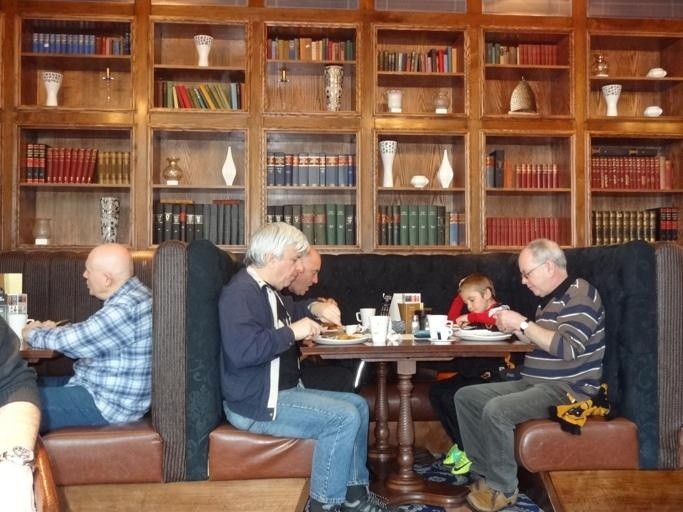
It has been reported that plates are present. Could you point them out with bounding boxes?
[428,339,455,342]
[307,326,369,345]
[450,324,475,329]
[456,329,513,342]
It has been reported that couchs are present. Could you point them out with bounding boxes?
[190,243,316,511]
[512,243,674,509]
[40,249,183,505]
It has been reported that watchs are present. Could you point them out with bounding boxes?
[520,317,531,335]
[0,445,36,473]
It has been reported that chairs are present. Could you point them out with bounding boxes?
[26,441,67,508]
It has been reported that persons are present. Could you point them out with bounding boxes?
[215,217,397,511]
[453,239,608,512]
[283,249,385,482]
[413,271,527,487]
[0,316,44,511]
[21,243,153,432]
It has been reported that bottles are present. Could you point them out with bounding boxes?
[591,53,611,76]
[29,217,52,245]
[162,157,183,185]
[221,146,236,185]
[437,149,454,188]
[411,315,420,334]
[432,91,450,114]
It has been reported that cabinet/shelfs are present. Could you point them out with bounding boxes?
[0,1,681,248]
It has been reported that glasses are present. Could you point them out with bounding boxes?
[519,260,549,280]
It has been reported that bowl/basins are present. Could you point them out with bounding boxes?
[409,176,429,188]
[645,68,667,79]
[643,106,663,118]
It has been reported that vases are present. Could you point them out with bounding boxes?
[218,143,239,188]
[599,78,623,118]
[591,46,614,81]
[319,62,347,114]
[29,213,55,245]
[188,31,215,69]
[384,84,410,116]
[158,154,188,187]
[429,87,451,117]
[409,175,430,190]
[643,66,669,80]
[93,196,120,246]
[375,139,400,190]
[642,105,664,120]
[436,148,456,190]
[39,67,67,110]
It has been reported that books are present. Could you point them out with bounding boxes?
[592,208,677,246]
[378,205,466,246]
[591,148,674,190]
[158,78,245,110]
[487,217,561,246]
[485,43,557,65]
[27,145,131,184]
[378,47,457,73]
[266,38,353,60]
[267,204,355,245]
[486,150,560,188]
[32,32,130,55]
[267,152,356,187]
[154,199,245,245]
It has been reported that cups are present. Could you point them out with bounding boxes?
[379,139,396,187]
[356,307,376,326]
[368,315,389,345]
[601,84,622,117]
[385,91,402,113]
[7,314,35,352]
[426,314,453,338]
[40,71,63,106]
[98,197,120,243]
[322,65,344,111]
[192,35,213,66]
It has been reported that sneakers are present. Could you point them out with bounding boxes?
[466,479,520,512]
[304,497,341,512]
[451,449,473,476]
[442,442,465,466]
[340,490,393,512]
